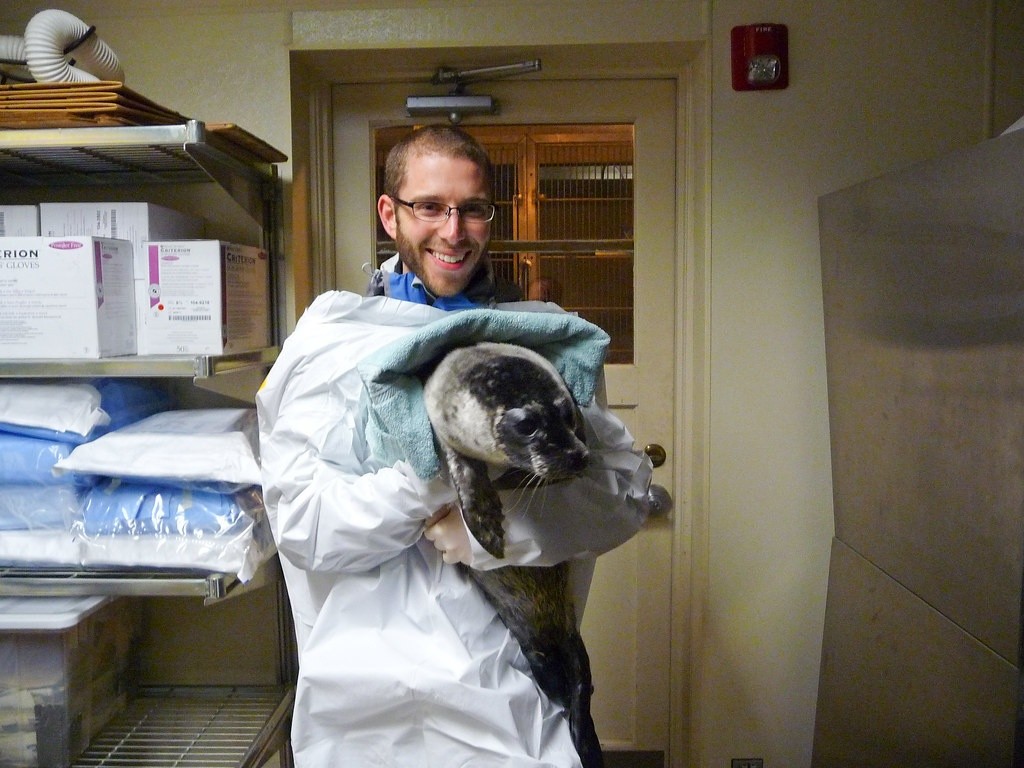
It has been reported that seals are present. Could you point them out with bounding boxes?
[423,343,605,768]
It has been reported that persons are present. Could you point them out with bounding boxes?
[258,124,651,768]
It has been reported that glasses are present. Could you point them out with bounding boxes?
[390,195,500,223]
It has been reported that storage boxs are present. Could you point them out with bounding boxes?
[40,202,203,326]
[0,205,40,237]
[142,240,273,354]
[0,595,139,768]
[0,236,138,359]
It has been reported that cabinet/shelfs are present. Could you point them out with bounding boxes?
[0,7,291,768]
